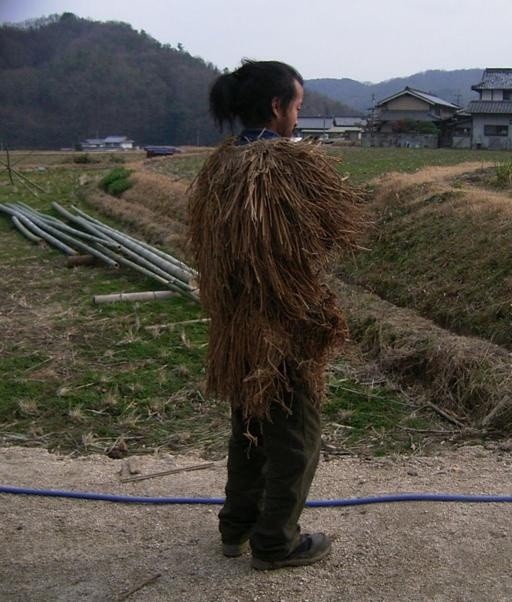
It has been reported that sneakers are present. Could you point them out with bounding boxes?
[251,532,332,571]
[221,540,250,559]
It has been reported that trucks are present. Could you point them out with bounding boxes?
[318,134,346,144]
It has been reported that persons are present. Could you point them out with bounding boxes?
[206,61,331,572]
